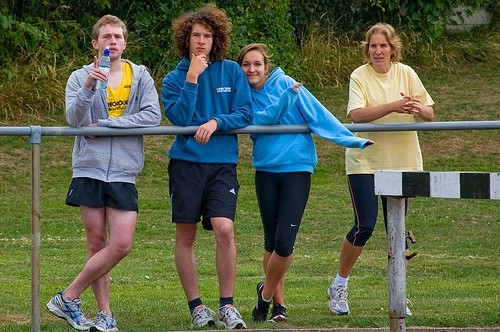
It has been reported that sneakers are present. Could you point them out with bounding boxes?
[216,303,247,330]
[251,280,271,323]
[327,283,350,315]
[190,304,215,327]
[89,310,119,332]
[405,297,413,317]
[269,302,286,322]
[45,292,95,331]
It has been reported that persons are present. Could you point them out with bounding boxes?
[161,4,254,330]
[239,44,375,323]
[45,14,161,332]
[328,22,435,317]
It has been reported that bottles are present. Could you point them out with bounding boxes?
[96,46,111,91]
